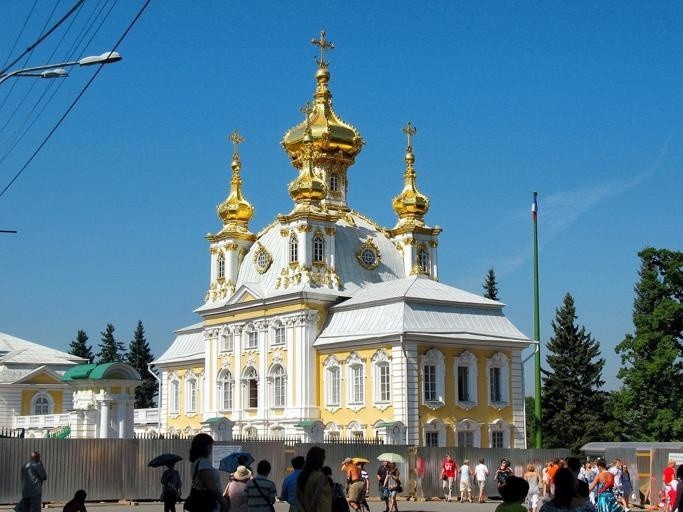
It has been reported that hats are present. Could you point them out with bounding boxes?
[342,457,352,464]
[234,466,251,480]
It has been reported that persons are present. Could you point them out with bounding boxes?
[542,456,634,512]
[441,453,457,502]
[21,452,48,512]
[188,434,229,512]
[280,446,350,512]
[341,457,370,512]
[496,459,513,486]
[63,490,87,512]
[473,458,489,503]
[495,475,529,512]
[223,456,277,512]
[458,460,473,503]
[525,464,543,512]
[376,461,401,512]
[663,459,683,512]
[160,463,182,511]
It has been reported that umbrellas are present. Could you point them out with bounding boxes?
[149,454,183,467]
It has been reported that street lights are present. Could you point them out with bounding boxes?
[0,67,68,80]
[0,51,120,86]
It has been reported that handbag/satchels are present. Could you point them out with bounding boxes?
[183,486,217,512]
[332,495,349,512]
